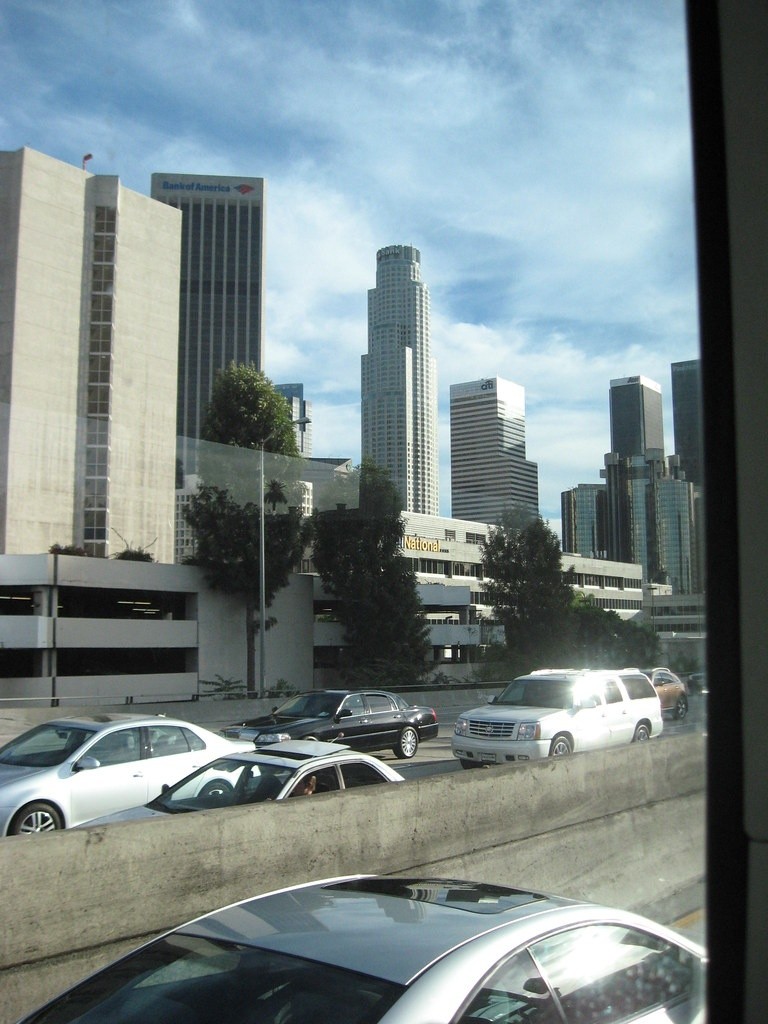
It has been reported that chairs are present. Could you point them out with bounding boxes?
[247,774,283,803]
[119,733,140,761]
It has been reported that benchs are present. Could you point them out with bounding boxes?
[149,730,191,757]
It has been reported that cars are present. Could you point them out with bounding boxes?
[217,690,439,761]
[69,741,407,829]
[1,714,260,840]
[7,876,707,1020]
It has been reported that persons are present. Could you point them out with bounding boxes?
[294,775,316,796]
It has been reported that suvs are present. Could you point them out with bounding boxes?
[451,670,665,771]
[623,667,689,721]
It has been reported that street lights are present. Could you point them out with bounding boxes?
[253,417,316,698]
